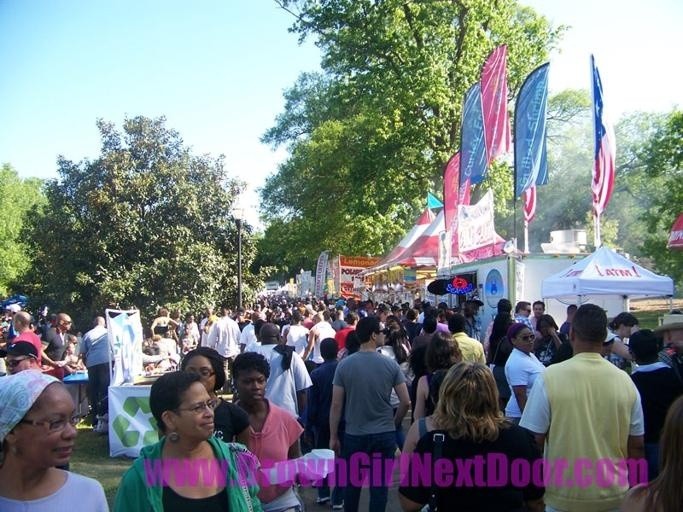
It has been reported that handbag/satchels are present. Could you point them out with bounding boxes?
[398,360,416,388]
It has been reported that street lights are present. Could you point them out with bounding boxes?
[229,203,243,314]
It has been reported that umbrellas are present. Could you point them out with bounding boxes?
[665,213,683,250]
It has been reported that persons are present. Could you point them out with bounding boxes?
[0,293,681,511]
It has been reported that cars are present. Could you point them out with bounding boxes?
[267,281,279,290]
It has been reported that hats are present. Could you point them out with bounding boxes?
[604,327,619,344]
[5,304,21,313]
[0,341,38,360]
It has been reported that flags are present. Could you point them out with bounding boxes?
[479,43,509,162]
[460,82,488,182]
[513,63,549,197]
[523,185,536,222]
[440,152,472,255]
[315,251,329,292]
[589,53,613,217]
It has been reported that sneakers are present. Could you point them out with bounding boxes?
[316,495,330,503]
[333,500,344,510]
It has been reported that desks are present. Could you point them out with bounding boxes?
[61,370,89,423]
[105,369,235,465]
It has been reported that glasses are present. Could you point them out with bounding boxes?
[518,333,536,341]
[378,329,386,334]
[21,412,81,435]
[4,357,31,367]
[197,369,216,380]
[170,398,218,414]
